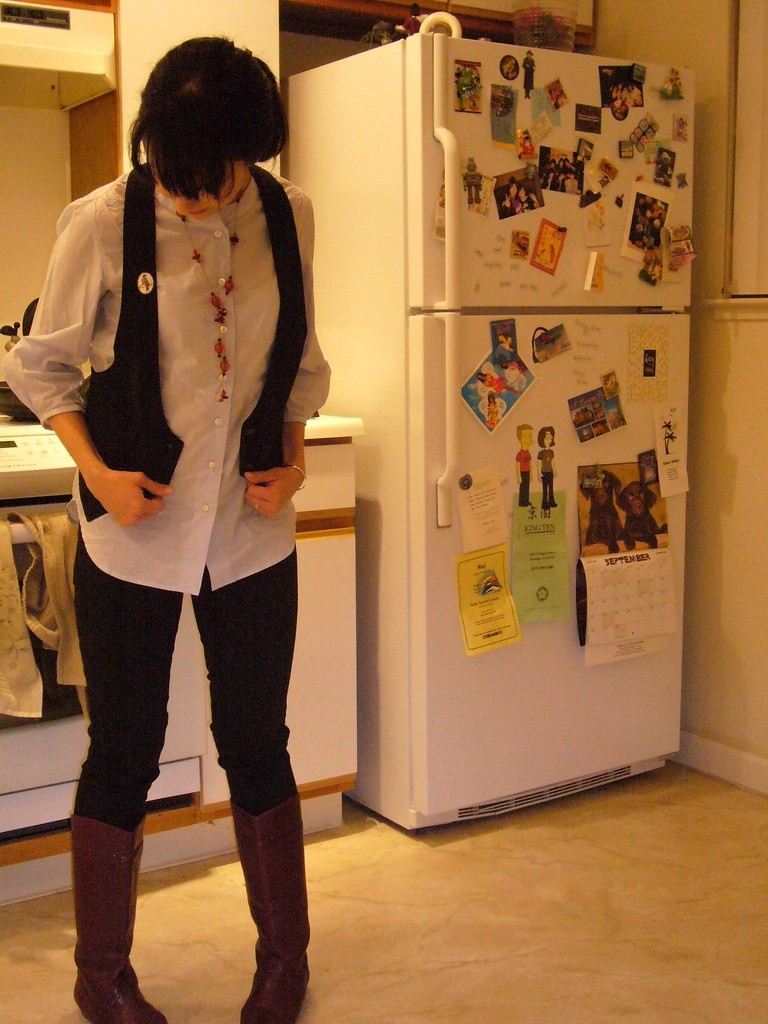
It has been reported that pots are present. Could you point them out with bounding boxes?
[0,381,39,419]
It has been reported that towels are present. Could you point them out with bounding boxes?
[0,511,78,718]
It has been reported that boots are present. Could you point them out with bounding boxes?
[71,814,168,1024]
[228,794,310,1024]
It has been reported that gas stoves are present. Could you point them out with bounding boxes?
[0,415,76,499]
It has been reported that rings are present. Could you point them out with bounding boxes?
[254,504,258,515]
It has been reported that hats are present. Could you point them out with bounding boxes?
[527,50,533,55]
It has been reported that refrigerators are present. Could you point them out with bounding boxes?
[287,34,692,830]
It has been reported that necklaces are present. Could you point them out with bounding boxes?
[176,194,241,402]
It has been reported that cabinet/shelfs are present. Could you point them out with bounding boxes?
[0,436,358,868]
[280,0,600,48]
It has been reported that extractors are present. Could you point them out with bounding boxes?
[0,1,117,112]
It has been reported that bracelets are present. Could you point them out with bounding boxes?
[290,465,307,490]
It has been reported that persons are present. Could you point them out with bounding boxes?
[677,118,688,142]
[610,82,643,107]
[523,50,535,99]
[571,376,625,441]
[7,37,333,1024]
[495,95,505,116]
[631,216,660,250]
[655,153,672,186]
[582,143,592,160]
[542,158,582,195]
[549,88,567,110]
[498,181,539,219]
[503,59,518,78]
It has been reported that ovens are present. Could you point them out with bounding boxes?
[0,501,208,842]
